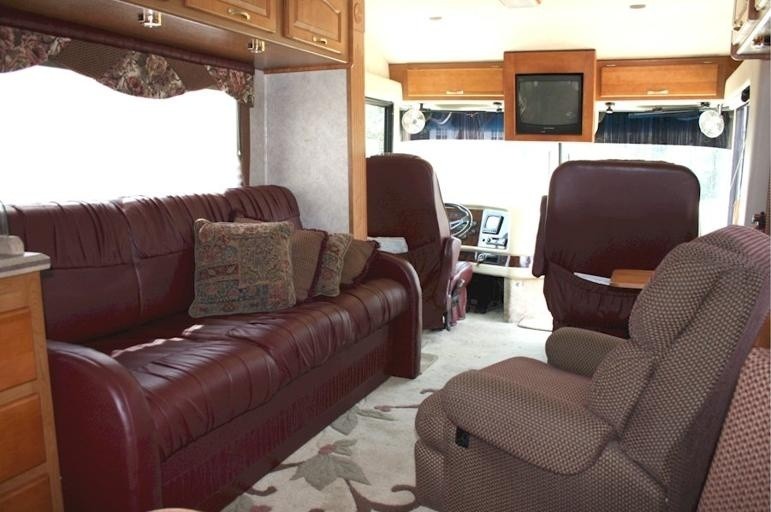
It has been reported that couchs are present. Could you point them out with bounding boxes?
[0,185,422,512]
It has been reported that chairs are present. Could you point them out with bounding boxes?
[532,160,700,339]
[414,224,770,512]
[366,152,473,332]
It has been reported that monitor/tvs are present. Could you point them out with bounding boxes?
[516,74,582,135]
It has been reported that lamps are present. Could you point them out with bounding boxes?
[137,8,162,29]
[698,104,724,139]
[248,37,265,54]
[401,104,425,135]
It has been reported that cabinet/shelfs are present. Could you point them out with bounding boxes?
[284,0,349,56]
[595,56,729,101]
[0,250,65,512]
[389,62,505,100]
[187,0,276,32]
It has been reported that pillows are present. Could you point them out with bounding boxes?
[188,209,381,319]
